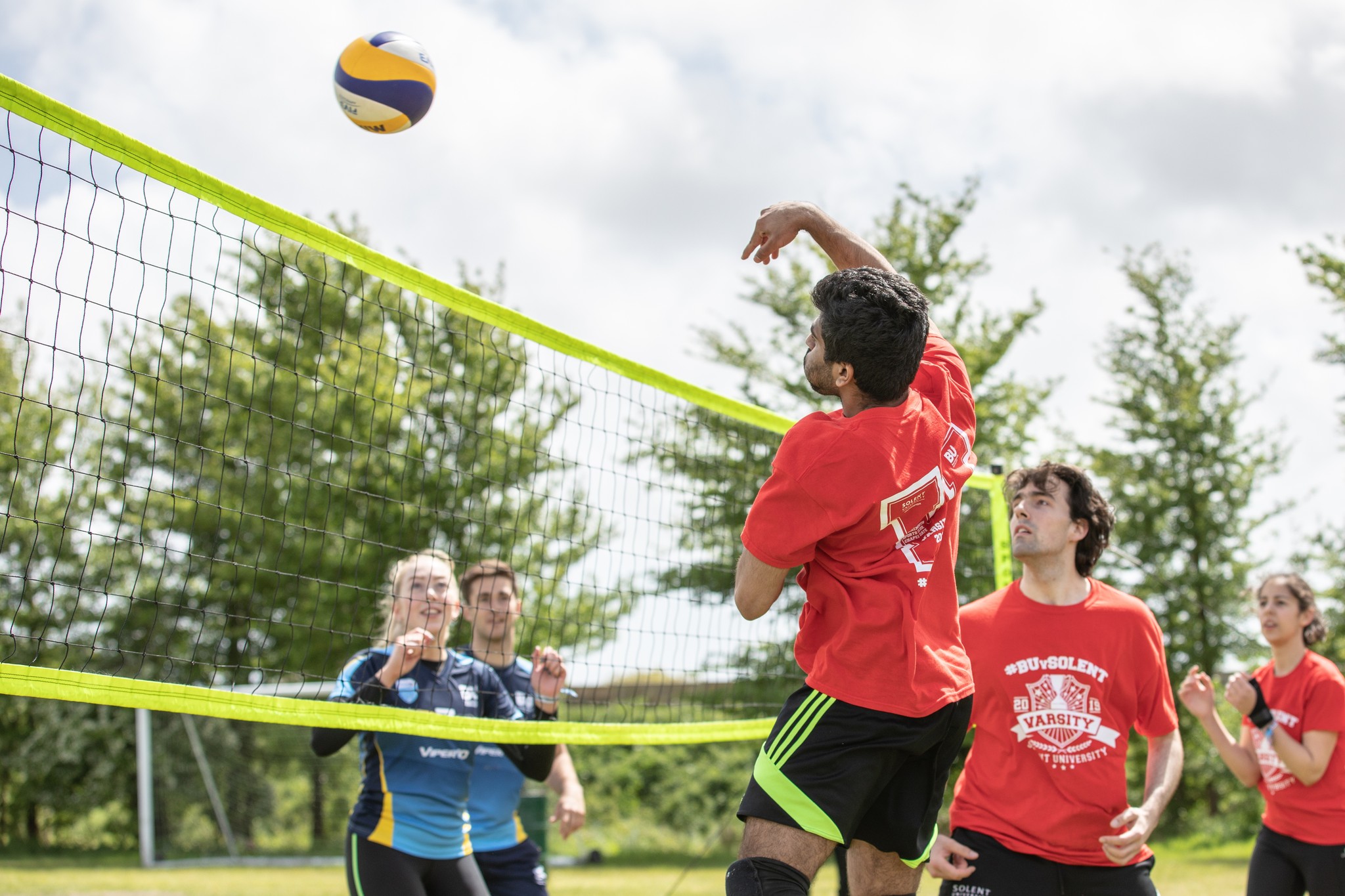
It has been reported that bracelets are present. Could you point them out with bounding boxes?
[531,687,577,703]
[1262,721,1278,737]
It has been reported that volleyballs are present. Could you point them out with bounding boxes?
[333,30,436,134]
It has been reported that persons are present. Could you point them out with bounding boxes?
[920,461,1185,896]
[311,549,590,896]
[725,202,976,896]
[1178,571,1345,896]
